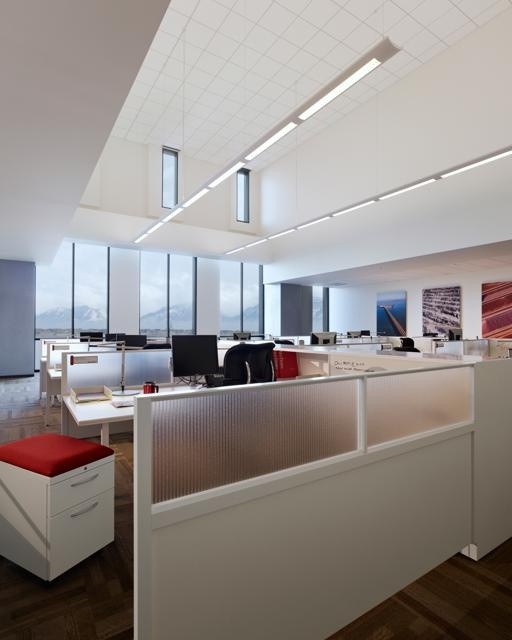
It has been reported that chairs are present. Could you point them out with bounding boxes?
[223,343,276,386]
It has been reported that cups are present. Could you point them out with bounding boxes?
[144,382,158,394]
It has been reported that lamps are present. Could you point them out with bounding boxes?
[226,124,511,257]
[129,1,402,247]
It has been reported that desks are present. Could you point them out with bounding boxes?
[48,368,62,380]
[63,382,199,446]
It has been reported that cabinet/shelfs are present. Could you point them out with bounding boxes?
[0,434,116,583]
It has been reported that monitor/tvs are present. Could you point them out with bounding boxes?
[310,333,337,345]
[347,332,363,339]
[234,333,252,340]
[448,329,463,342]
[116,335,148,352]
[79,331,103,344]
[170,334,219,387]
[106,333,125,345]
